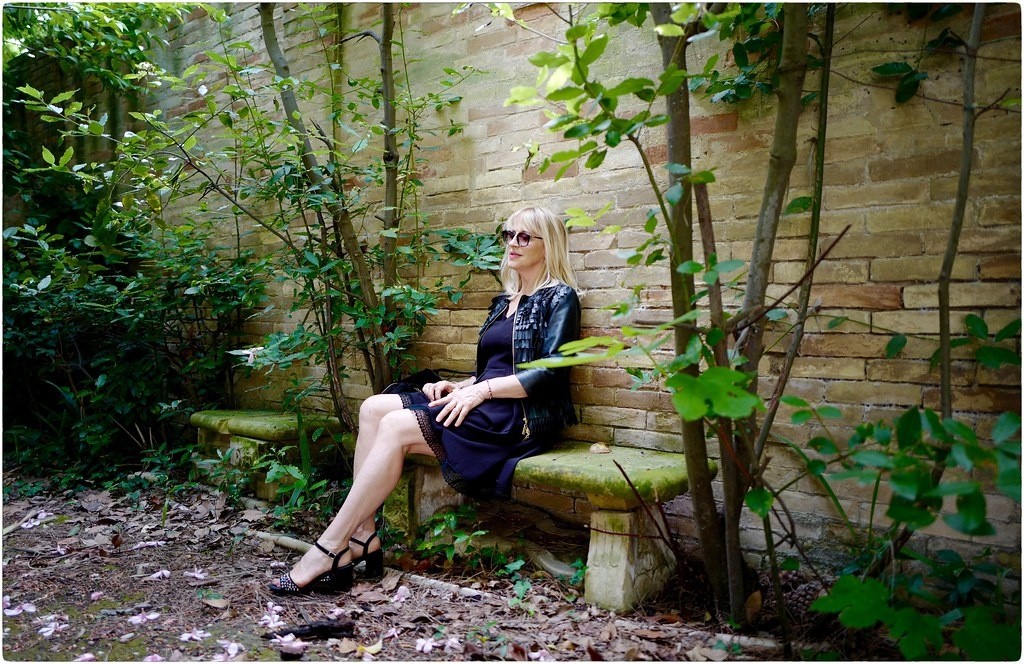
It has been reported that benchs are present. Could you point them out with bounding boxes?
[382,439,718,615]
[190,408,346,504]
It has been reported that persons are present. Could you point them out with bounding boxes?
[267,204,582,594]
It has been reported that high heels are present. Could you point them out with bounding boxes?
[267,542,355,594]
[350,532,384,579]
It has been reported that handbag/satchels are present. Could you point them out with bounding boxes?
[381,368,476,394]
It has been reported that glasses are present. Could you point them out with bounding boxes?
[502,230,543,247]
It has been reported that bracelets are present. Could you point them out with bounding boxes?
[486,379,494,400]
